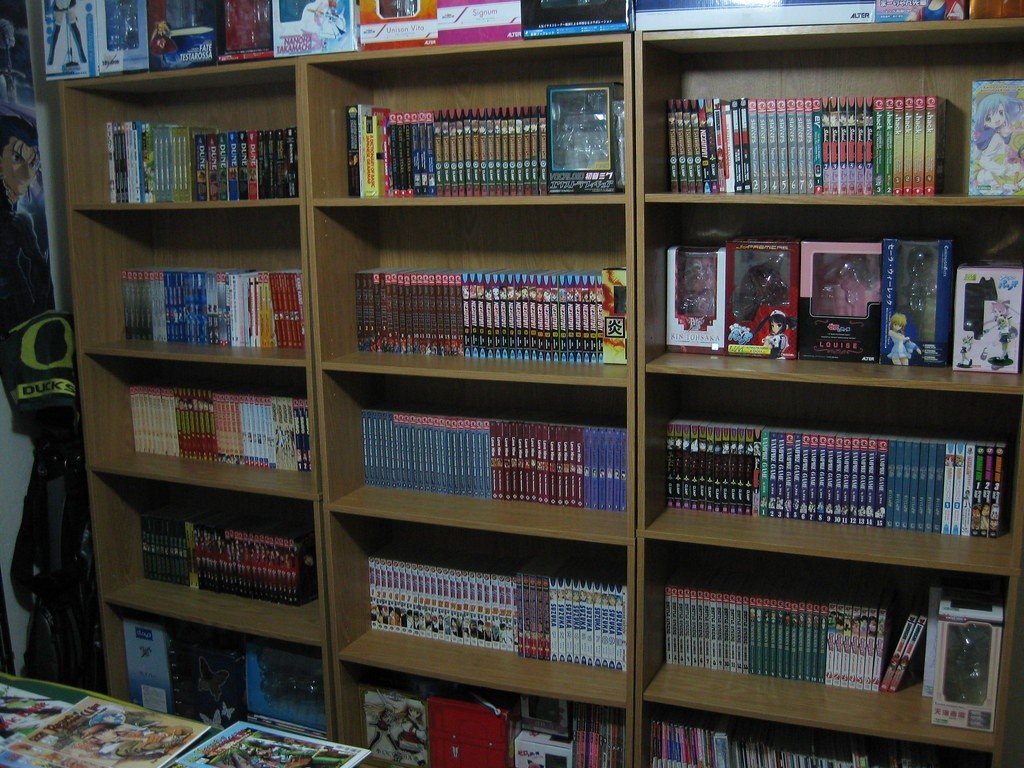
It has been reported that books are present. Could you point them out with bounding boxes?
[2,81,1022,766]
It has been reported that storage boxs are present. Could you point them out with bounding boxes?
[546,84,623,195]
[437,0,523,45]
[41,0,100,83]
[797,237,883,363]
[272,0,362,59]
[148,0,220,71]
[952,258,1023,374]
[724,235,802,361]
[930,619,1002,733]
[968,78,1024,196]
[521,0,630,40]
[96,0,150,73]
[217,0,274,63]
[666,244,727,354]
[878,235,956,368]
[360,0,438,52]
[631,0,877,32]
[123,617,574,768]
[875,0,967,23]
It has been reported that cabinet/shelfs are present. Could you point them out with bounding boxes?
[633,30,1024,768]
[295,32,635,768]
[56,56,338,743]
